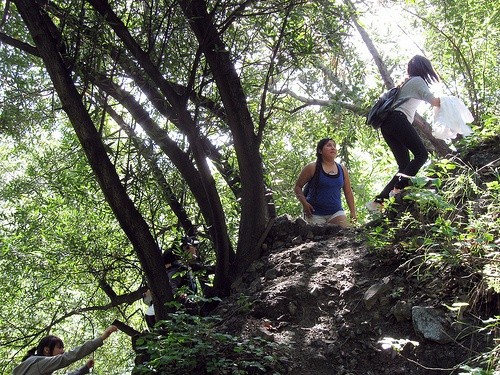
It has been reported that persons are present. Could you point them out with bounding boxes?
[364,54,465,214]
[12,325,118,375]
[142,236,202,333]
[293,137,357,228]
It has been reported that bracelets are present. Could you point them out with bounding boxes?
[302,200,308,205]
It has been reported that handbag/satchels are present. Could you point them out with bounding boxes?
[304,162,321,199]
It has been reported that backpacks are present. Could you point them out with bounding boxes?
[365,76,415,130]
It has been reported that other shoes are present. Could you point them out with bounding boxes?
[389,188,401,197]
[365,202,380,217]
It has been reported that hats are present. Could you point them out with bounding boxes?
[175,237,200,248]
